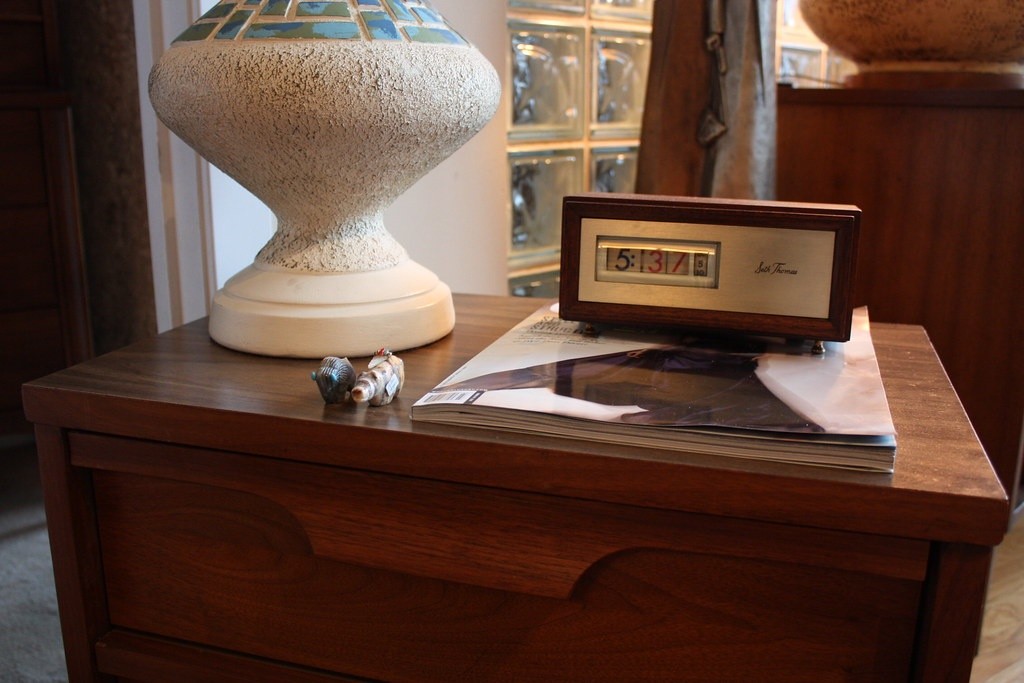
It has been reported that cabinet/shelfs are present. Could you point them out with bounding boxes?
[769,81,1024,516]
[21,291,1015,683]
[0,0,103,436]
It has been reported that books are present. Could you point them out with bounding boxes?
[408,305,897,475]
